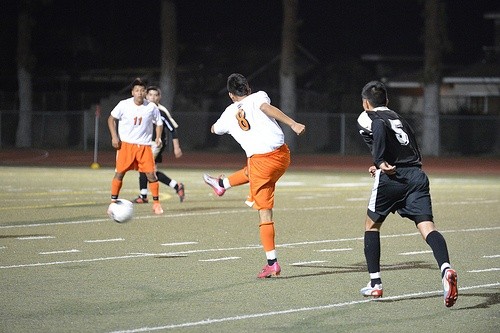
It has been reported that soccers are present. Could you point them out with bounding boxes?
[106,199,136,224]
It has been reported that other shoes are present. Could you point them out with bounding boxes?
[153,203,163,215]
[131,195,148,203]
[175,182,185,203]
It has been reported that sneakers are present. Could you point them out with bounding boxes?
[202,172,226,197]
[441,269,458,308]
[258,261,281,278]
[361,281,383,299]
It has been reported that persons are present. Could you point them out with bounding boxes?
[357,81,459,308]
[202,74,305,278]
[108,77,163,214]
[130,87,185,204]
